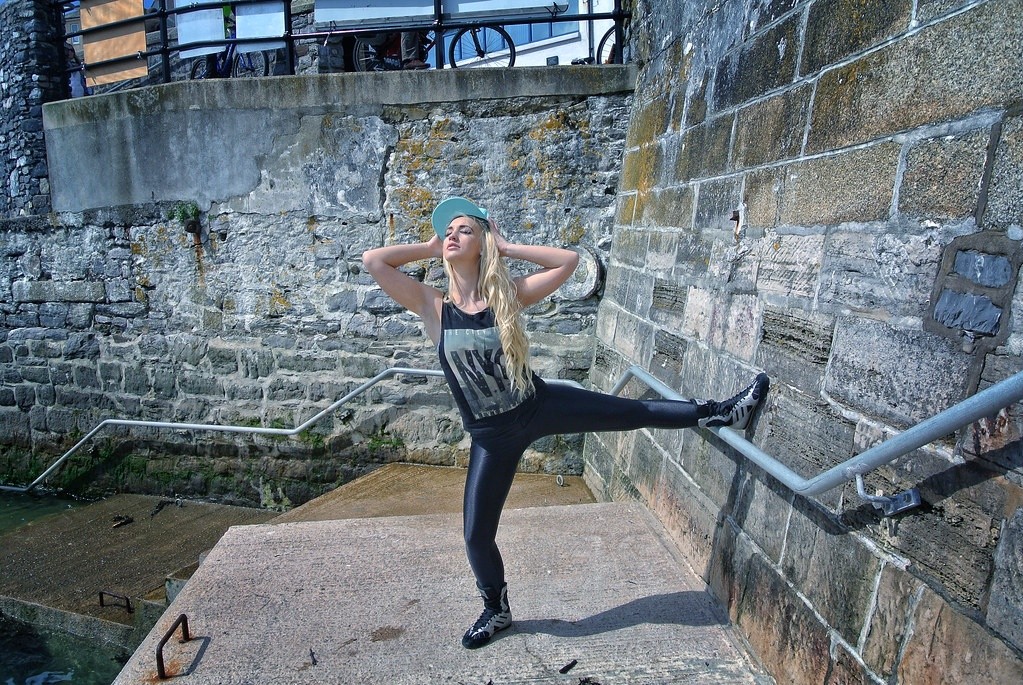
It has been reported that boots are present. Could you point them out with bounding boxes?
[462,580,512,649]
[690,372,770,431]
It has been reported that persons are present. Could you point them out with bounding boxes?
[363,196,769,648]
[400,31,431,69]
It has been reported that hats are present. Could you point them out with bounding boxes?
[432,197,497,244]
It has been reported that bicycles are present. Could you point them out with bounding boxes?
[353,26,515,67]
[189,24,269,77]
[570,26,615,66]
